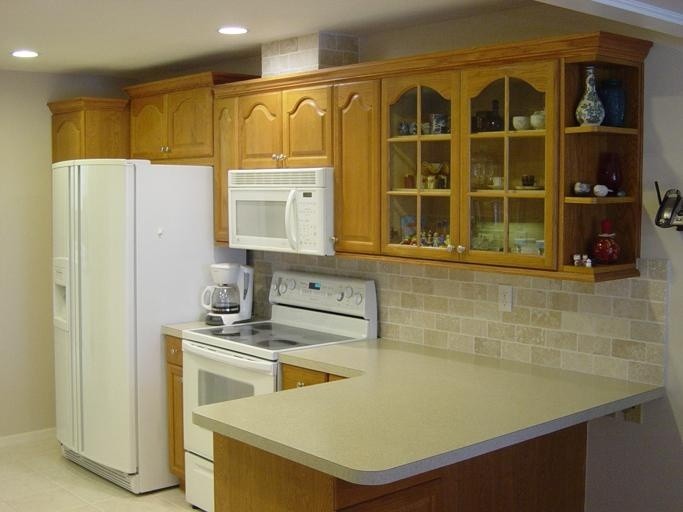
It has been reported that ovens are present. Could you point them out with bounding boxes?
[179,339,277,511]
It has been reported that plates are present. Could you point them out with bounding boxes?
[514,186,543,189]
[487,184,509,189]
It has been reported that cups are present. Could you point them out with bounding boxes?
[522,175,536,185]
[492,176,504,185]
[404,174,414,188]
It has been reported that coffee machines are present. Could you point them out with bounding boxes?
[199,263,253,326]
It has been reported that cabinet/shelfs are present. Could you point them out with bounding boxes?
[45,94,128,165]
[164,327,185,495]
[333,60,382,261]
[558,31,654,284]
[118,70,263,165]
[277,363,347,392]
[381,29,558,279]
[239,68,332,168]
[213,80,240,245]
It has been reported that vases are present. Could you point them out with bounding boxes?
[596,152,623,196]
[573,65,606,126]
[602,77,625,126]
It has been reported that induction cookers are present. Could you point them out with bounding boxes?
[190,321,356,352]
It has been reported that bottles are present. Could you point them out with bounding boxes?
[480,100,504,131]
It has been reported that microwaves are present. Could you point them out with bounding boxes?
[227,167,334,258]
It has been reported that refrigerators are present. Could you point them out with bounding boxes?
[49,155,213,493]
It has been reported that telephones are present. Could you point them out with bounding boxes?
[655,181,683,228]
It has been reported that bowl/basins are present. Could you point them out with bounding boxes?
[398,113,544,135]
